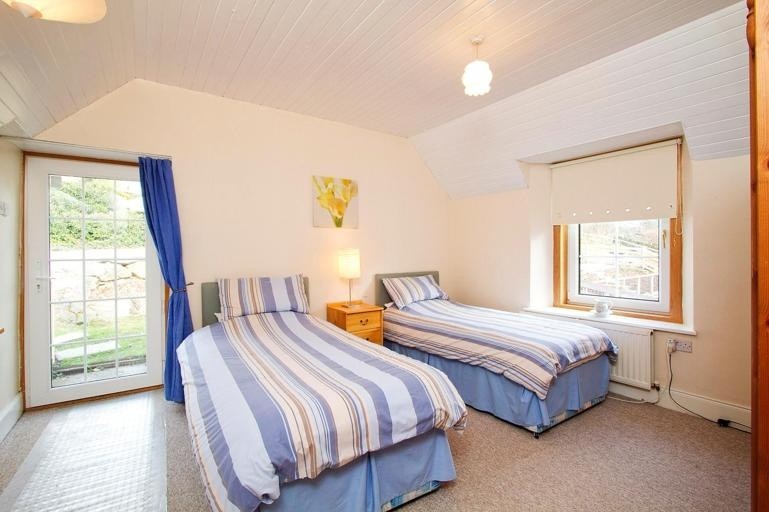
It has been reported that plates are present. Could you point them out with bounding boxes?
[590,310,613,318]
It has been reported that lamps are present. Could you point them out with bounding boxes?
[339,248,361,308]
[458,37,493,98]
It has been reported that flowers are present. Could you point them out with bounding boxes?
[314,176,358,227]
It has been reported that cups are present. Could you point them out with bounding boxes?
[594,299,613,313]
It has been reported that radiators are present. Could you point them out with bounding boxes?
[524,308,654,391]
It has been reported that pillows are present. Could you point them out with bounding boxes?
[381,275,447,309]
[214,276,311,321]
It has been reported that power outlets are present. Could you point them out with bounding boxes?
[666,340,692,352]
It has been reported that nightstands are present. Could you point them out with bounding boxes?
[328,300,384,347]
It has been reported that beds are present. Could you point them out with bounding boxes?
[175,275,467,512]
[374,271,618,439]
